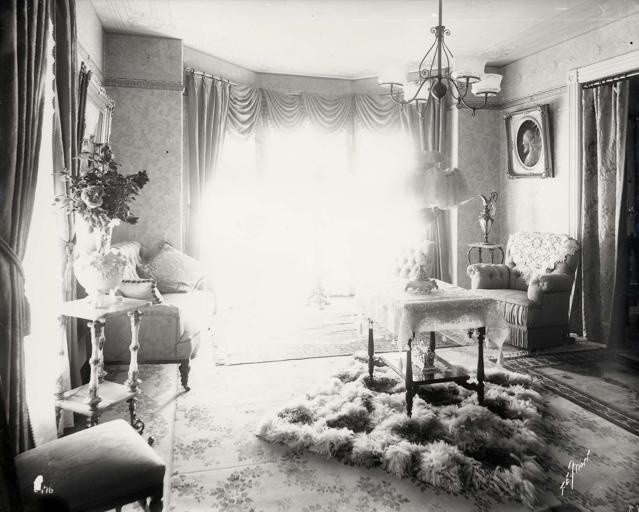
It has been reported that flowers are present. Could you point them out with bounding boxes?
[51,133,150,231]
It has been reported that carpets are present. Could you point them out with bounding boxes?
[254,352,545,509]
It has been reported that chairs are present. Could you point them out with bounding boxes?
[466,230,578,350]
[2,244,165,510]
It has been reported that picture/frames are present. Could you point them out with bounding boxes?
[504,104,553,180]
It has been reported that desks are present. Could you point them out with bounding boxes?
[48,295,147,434]
[366,277,495,418]
[467,243,505,266]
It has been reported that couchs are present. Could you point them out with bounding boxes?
[74,239,217,393]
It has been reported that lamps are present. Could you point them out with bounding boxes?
[378,0,507,116]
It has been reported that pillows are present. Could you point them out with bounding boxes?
[121,278,165,305]
[145,243,207,290]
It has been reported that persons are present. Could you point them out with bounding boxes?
[521,129,541,167]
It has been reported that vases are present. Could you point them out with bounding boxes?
[73,222,130,310]
